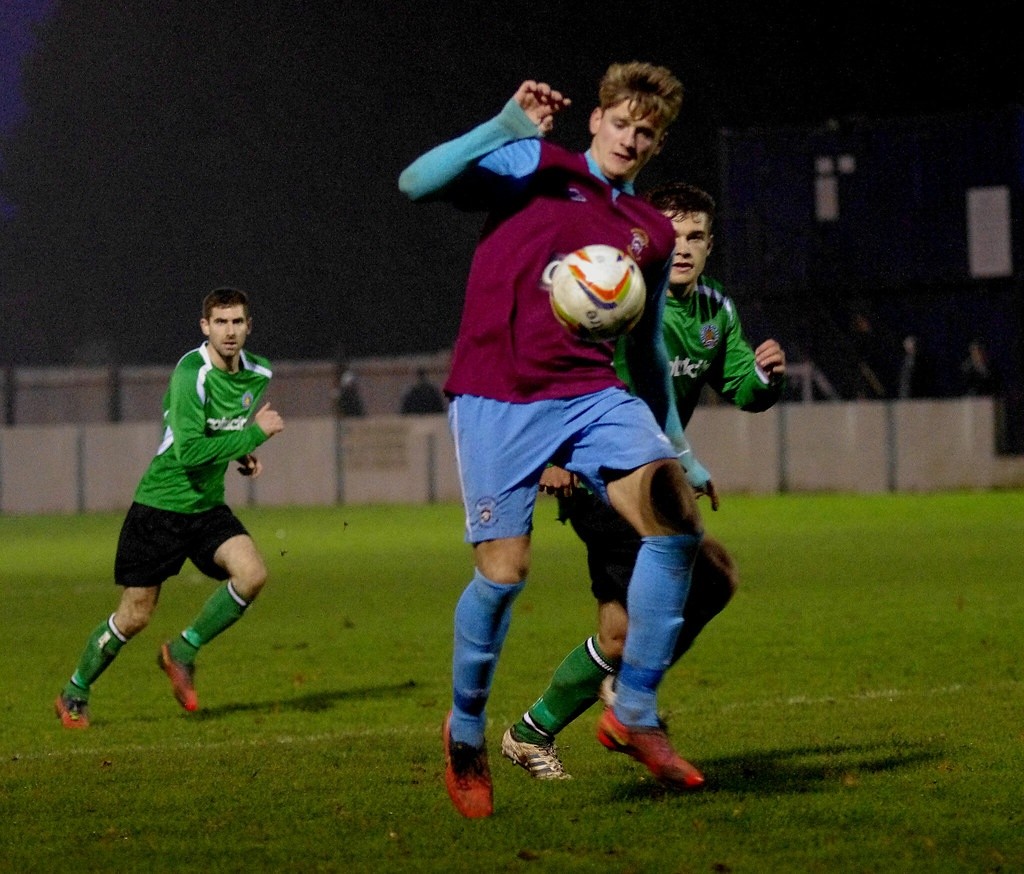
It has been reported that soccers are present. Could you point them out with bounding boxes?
[548,242,647,343]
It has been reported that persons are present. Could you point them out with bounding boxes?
[330,352,367,417]
[397,63,787,821]
[56,287,284,728]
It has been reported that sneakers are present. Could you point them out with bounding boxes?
[441,709,493,819]
[501,723,574,783]
[158,642,198,711]
[55,694,90,730]
[600,671,618,708]
[597,708,705,789]
